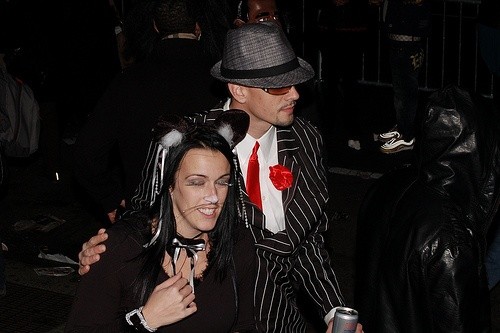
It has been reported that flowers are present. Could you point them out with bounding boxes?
[269,164,293,191]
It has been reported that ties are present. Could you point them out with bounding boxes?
[246,141,263,213]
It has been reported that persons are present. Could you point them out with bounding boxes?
[70,109,258,333]
[0,1,500,333]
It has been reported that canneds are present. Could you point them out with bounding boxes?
[332,307,359,333]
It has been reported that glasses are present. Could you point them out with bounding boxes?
[261,86,292,95]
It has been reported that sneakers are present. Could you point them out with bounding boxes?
[379,135,414,154]
[377,131,402,142]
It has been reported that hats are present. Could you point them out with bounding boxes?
[210,19,315,88]
